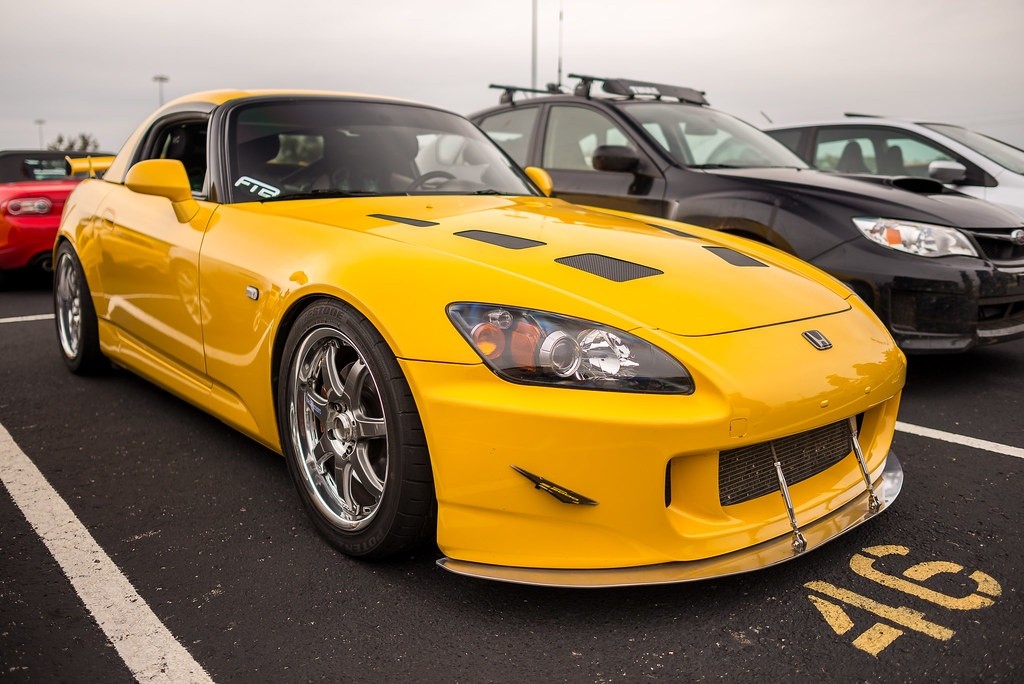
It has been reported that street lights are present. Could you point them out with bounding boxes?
[35,118,45,149]
[153,75,169,108]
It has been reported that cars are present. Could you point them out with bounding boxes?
[427,71,1024,353]
[0,150,117,291]
[759,111,1024,221]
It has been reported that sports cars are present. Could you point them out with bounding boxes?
[49,86,910,589]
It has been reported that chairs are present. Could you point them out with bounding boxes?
[835,141,914,176]
[324,112,408,193]
[169,126,207,176]
[237,110,281,200]
[549,127,593,171]
